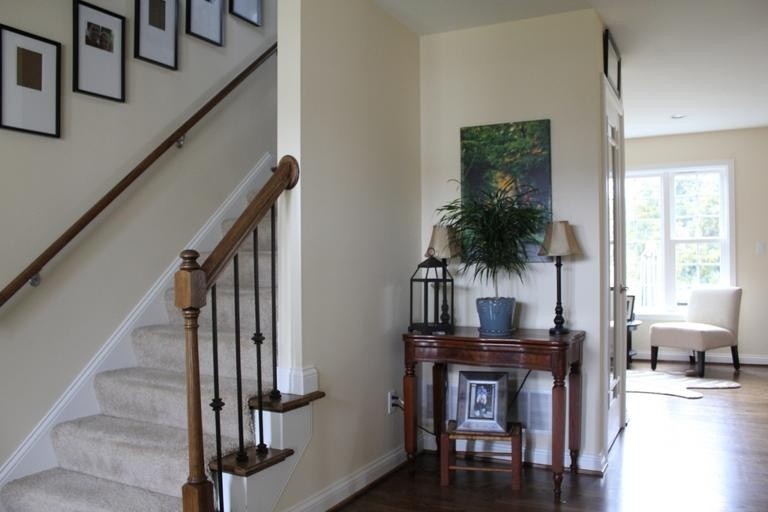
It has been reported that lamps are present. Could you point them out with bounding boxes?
[539,221,582,335]
[425,224,460,329]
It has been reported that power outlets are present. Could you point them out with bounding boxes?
[387,390,398,414]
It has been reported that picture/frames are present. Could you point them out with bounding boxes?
[0,23,62,138]
[72,0,126,103]
[626,295,635,322]
[133,0,179,72]
[229,0,261,27]
[456,371,509,434]
[185,0,225,47]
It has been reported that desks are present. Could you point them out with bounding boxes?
[403,326,586,494]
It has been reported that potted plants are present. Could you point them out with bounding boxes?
[435,175,550,336]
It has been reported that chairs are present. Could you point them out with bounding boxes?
[650,286,742,377]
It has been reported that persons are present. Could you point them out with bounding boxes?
[474,386,487,418]
[85,22,113,52]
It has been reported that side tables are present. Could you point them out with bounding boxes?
[610,320,643,369]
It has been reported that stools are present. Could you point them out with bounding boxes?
[440,420,522,491]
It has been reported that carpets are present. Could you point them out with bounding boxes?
[610,368,741,398]
